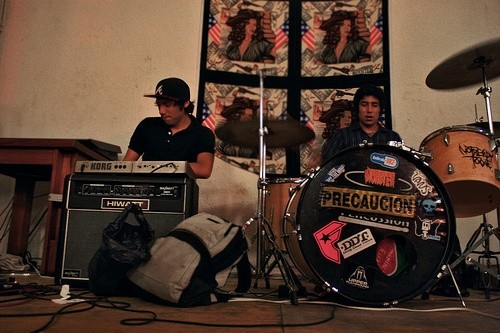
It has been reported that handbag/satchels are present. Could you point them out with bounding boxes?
[87,204,154,298]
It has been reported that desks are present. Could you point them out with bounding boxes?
[0,137,109,276]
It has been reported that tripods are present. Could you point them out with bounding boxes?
[241,70,309,305]
[421,65,500,300]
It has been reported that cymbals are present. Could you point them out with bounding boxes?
[425,37,500,91]
[215,117,315,150]
[466,122,500,140]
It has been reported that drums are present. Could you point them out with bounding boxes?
[284,145,456,310]
[420,125,500,218]
[265,173,308,253]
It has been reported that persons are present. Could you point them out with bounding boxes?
[122,78,215,181]
[320,85,404,165]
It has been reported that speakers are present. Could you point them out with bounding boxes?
[55,172,200,289]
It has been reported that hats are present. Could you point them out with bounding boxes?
[144,78,190,102]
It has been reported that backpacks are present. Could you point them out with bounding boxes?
[122,213,252,308]
